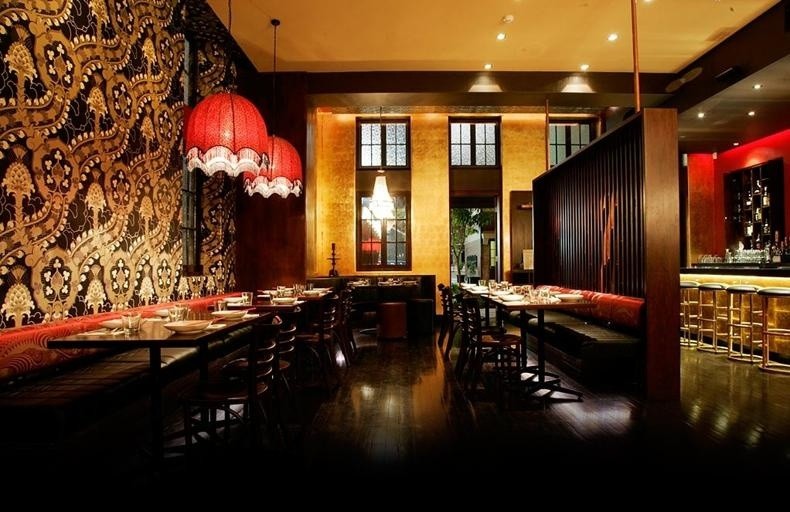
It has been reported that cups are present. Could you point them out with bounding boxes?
[697,250,766,265]
[120,311,142,335]
[213,300,228,321]
[168,305,189,322]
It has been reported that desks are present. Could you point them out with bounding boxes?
[352,275,437,342]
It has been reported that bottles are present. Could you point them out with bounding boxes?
[737,180,790,264]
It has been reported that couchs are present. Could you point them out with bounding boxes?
[511,286,644,385]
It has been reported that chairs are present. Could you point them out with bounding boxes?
[0,279,359,451]
[437,274,586,400]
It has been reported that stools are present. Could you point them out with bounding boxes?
[678,280,701,349]
[759,285,788,377]
[697,281,736,356]
[726,284,772,367]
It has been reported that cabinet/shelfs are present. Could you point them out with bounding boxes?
[723,155,786,253]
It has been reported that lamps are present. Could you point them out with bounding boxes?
[173,0,272,179]
[242,16,305,198]
[370,105,397,206]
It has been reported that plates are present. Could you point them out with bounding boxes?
[225,284,330,305]
[211,311,248,318]
[99,319,147,329]
[349,277,417,286]
[163,320,213,334]
[458,279,583,304]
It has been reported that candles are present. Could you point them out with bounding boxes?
[332,242,335,250]
